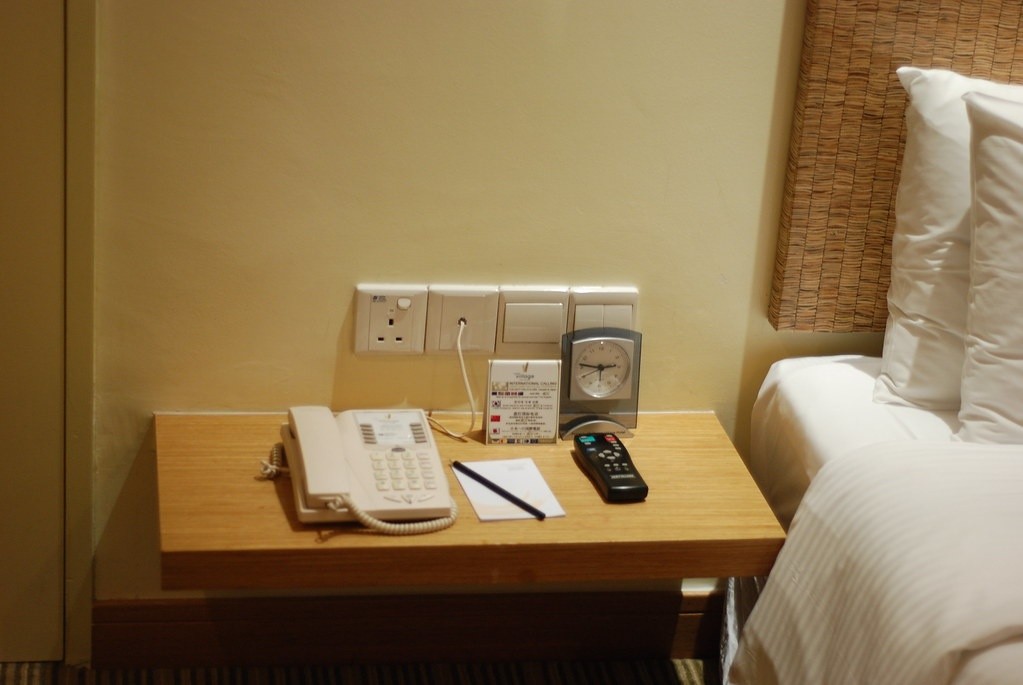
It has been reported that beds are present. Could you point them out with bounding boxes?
[729,0,1023,685]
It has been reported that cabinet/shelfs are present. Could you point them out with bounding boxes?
[153,411,787,595]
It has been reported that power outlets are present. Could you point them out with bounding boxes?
[353,283,426,354]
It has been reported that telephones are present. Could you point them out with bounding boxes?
[279,404,455,527]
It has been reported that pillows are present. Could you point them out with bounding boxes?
[950,92,1023,449]
[872,66,1023,410]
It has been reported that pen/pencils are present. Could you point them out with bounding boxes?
[453,460,545,522]
